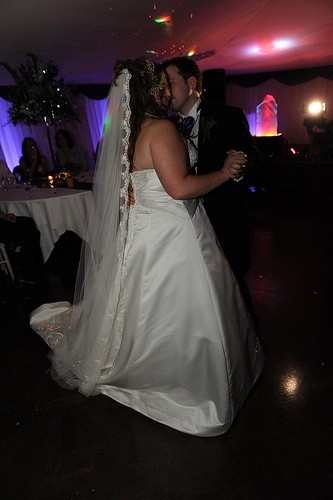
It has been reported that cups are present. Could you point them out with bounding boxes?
[67,178,74,187]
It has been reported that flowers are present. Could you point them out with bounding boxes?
[200,116,217,142]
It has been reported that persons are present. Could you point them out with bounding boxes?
[19,137,47,173]
[0,209,41,288]
[161,57,261,330]
[55,129,96,190]
[30,56,265,438]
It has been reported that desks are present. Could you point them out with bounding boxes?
[0,186,92,266]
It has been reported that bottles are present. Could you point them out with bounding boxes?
[23,182,32,199]
[0,176,15,192]
